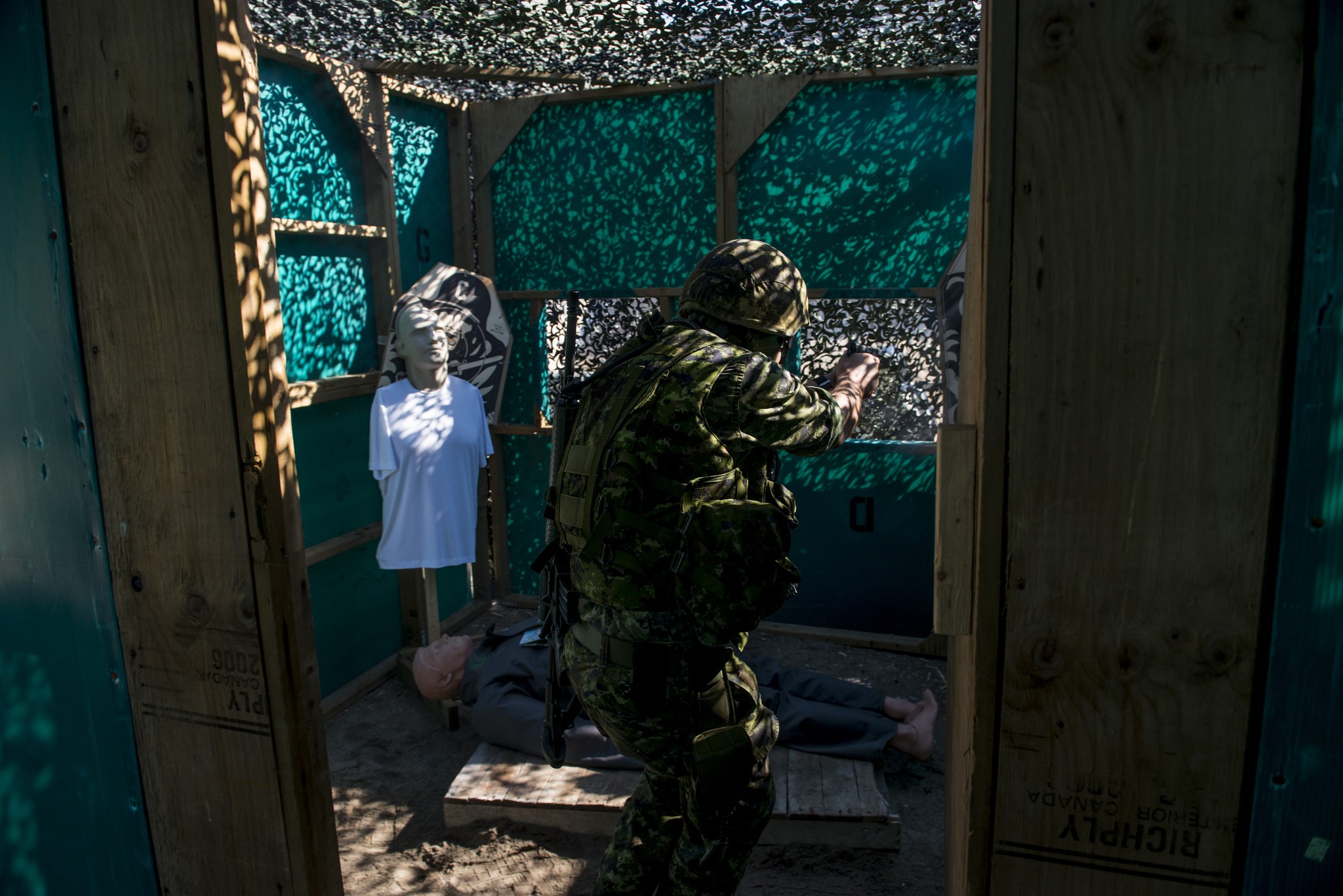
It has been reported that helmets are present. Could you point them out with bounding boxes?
[678,239,811,337]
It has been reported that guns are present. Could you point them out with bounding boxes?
[846,342,883,359]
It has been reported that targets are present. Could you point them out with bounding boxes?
[377,262,516,428]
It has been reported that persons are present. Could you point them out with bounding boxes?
[412,617,937,768]
[368,306,494,570]
[559,239,880,896]
